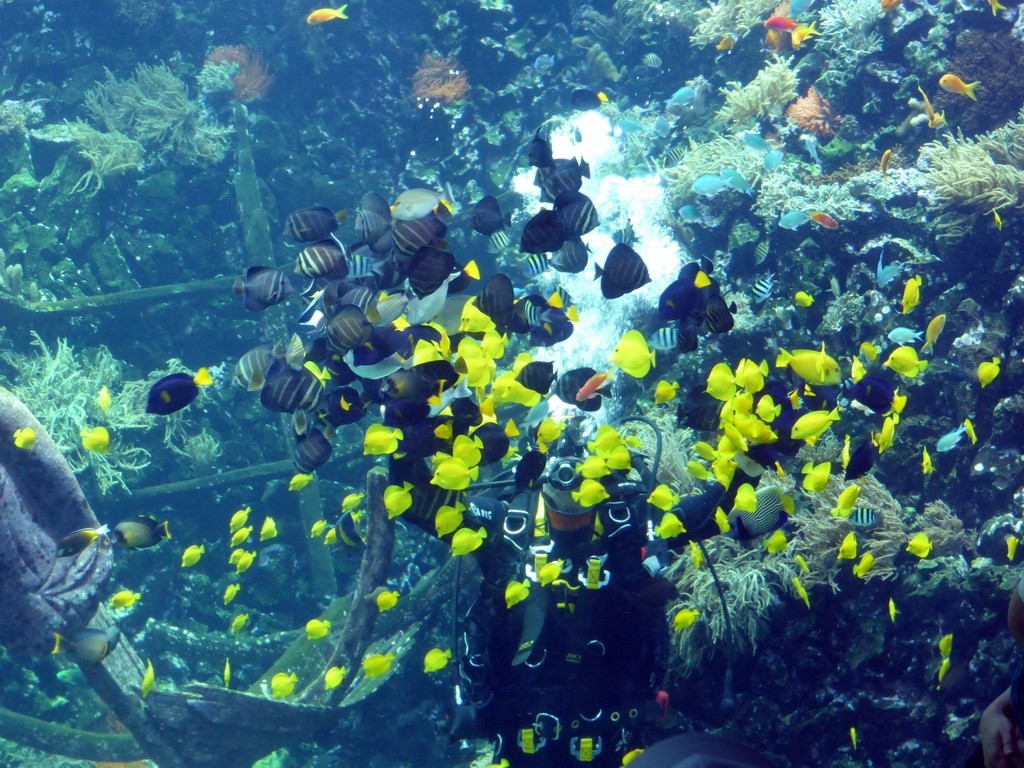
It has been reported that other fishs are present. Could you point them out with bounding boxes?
[17,2,1024,707]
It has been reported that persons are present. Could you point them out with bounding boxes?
[371,382,821,768]
